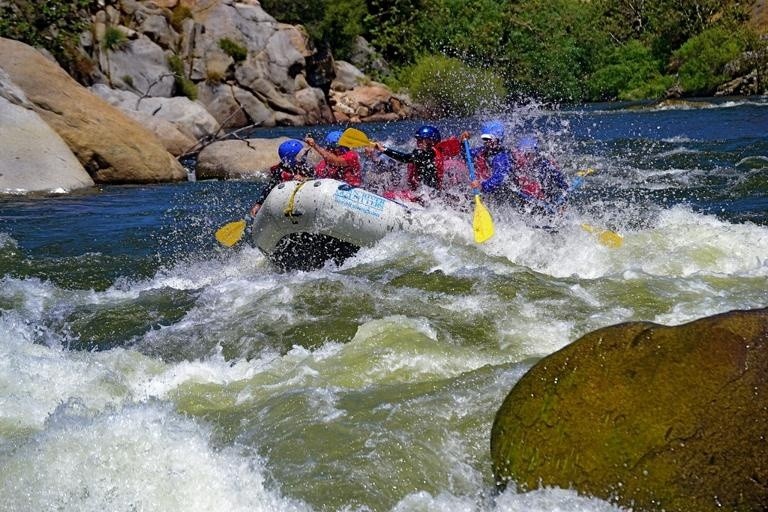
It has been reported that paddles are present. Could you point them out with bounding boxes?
[215,218,248,247]
[338,128,411,157]
[462,138,495,243]
[575,168,594,177]
[581,224,622,247]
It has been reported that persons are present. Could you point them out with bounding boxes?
[249,138,318,216]
[363,116,569,233]
[294,130,363,189]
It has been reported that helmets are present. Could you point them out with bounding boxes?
[325,131,344,146]
[482,122,505,141]
[414,125,441,143]
[278,140,303,160]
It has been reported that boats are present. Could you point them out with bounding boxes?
[250,178,525,270]
